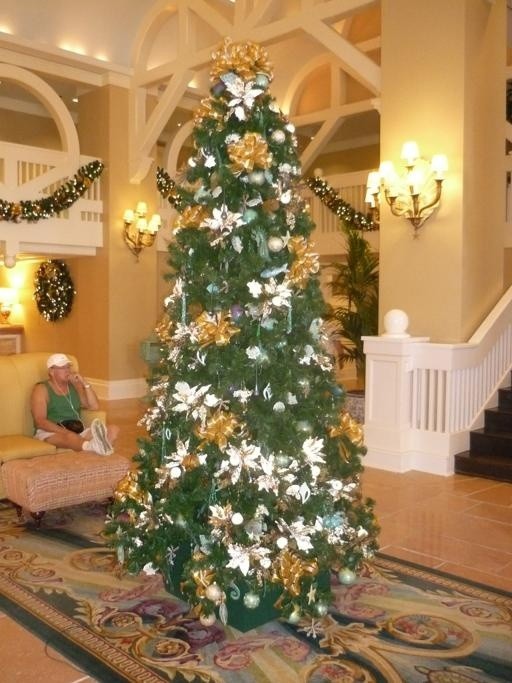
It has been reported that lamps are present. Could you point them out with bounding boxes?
[380,141,447,238]
[381,308,411,338]
[122,203,162,262]
[0,288,18,323]
[364,171,380,231]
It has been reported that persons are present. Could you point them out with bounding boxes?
[30,353,114,456]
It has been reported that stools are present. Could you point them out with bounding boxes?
[0,451,133,530]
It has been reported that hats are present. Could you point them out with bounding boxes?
[46,353,72,369]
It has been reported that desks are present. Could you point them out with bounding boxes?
[0,325,24,356]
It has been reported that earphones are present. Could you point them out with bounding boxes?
[52,369,55,373]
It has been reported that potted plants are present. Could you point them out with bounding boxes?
[319,222,379,424]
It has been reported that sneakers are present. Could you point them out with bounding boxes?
[90,418,114,456]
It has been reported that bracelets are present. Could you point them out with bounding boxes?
[84,384,91,388]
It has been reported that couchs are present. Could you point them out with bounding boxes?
[0,352,107,465]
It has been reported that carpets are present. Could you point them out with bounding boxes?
[0,498,512,683]
